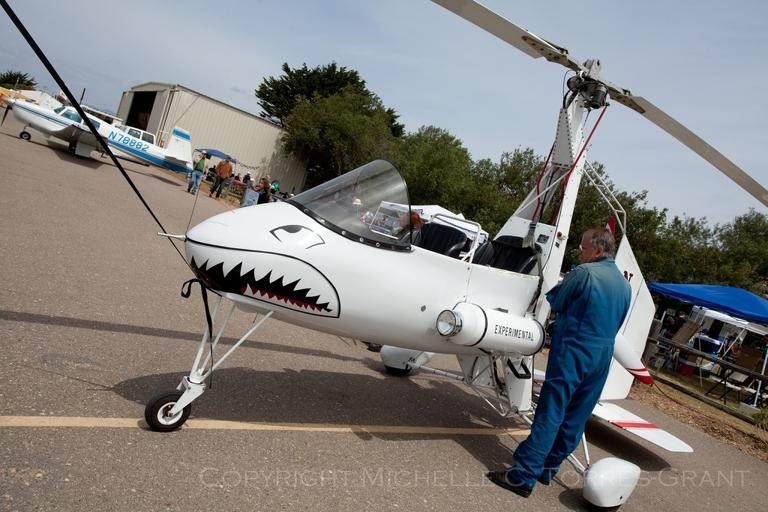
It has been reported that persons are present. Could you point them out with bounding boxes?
[485,224,633,499]
[394,210,424,247]
[187,150,296,205]
[652,300,768,388]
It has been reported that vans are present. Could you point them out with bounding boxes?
[110,123,155,166]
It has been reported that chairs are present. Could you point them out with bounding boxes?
[490,235,543,274]
[412,222,468,259]
[641,319,661,367]
[658,319,700,374]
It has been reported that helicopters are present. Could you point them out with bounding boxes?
[143,1,767,510]
[1,88,194,176]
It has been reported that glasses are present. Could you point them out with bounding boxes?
[579,245,597,252]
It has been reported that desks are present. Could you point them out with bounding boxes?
[658,335,768,407]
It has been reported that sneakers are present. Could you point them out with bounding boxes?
[488,463,550,497]
